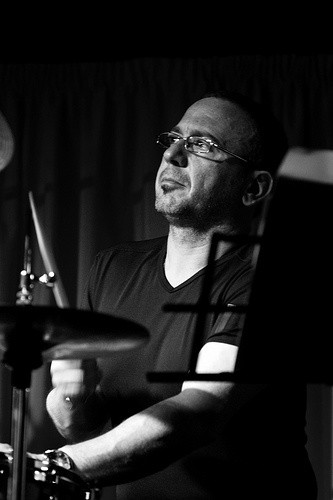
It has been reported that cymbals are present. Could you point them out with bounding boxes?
[0,304,151,361]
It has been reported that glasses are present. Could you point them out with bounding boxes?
[156,133,249,165]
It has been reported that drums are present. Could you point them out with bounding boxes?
[0,445,100,500]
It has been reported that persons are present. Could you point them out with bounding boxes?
[0,92,319,500]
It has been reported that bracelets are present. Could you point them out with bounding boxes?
[43,450,89,485]
